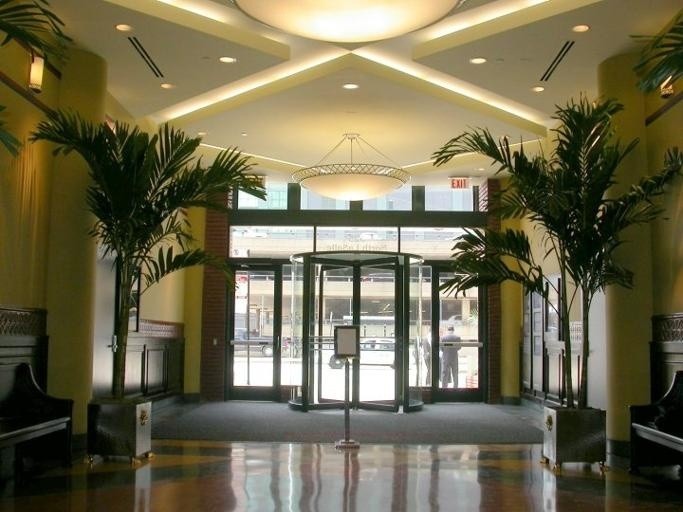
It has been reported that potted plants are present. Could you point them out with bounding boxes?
[433,95,683,469]
[26,105,268,468]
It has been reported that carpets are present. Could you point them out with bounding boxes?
[146,394,552,445]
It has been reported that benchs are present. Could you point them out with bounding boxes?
[1,307,74,475]
[627,311,683,484]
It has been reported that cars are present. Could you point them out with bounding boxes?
[328,339,417,370]
[234,328,288,357]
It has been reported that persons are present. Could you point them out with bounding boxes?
[441,327,461,388]
[422,328,433,385]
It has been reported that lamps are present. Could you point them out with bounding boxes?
[28,47,49,94]
[232,0,463,45]
[290,132,412,202]
